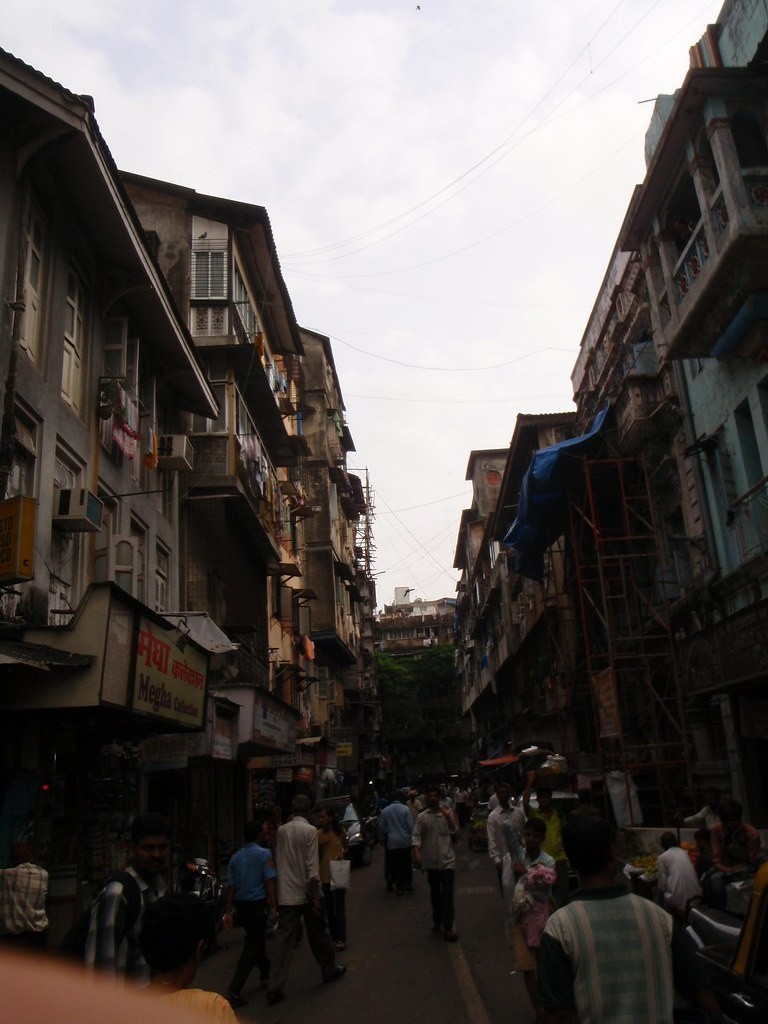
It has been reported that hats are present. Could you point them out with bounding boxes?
[660,831,678,846]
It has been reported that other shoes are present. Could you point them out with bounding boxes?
[337,962,350,976]
[265,989,287,1004]
[431,926,443,936]
[331,940,346,952]
[225,992,246,1008]
[445,929,459,940]
[397,886,415,897]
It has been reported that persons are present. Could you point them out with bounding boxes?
[412,788,460,942]
[317,806,347,951]
[63,814,198,988]
[487,784,526,901]
[0,768,768,1024]
[267,795,347,1000]
[223,821,277,1006]
[375,791,414,895]
[538,816,699,1024]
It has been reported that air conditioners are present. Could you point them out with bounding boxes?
[52,488,105,533]
[157,434,194,472]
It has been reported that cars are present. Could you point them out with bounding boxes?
[309,800,373,868]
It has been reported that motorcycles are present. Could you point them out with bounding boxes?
[179,856,227,956]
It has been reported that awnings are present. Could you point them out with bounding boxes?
[280,664,320,690]
[335,561,353,580]
[348,585,363,601]
[287,434,313,458]
[278,480,314,523]
[157,611,238,653]
[296,736,326,744]
[281,563,317,604]
[279,397,295,417]
[479,755,519,767]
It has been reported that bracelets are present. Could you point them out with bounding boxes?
[311,896,320,900]
[225,910,232,914]
